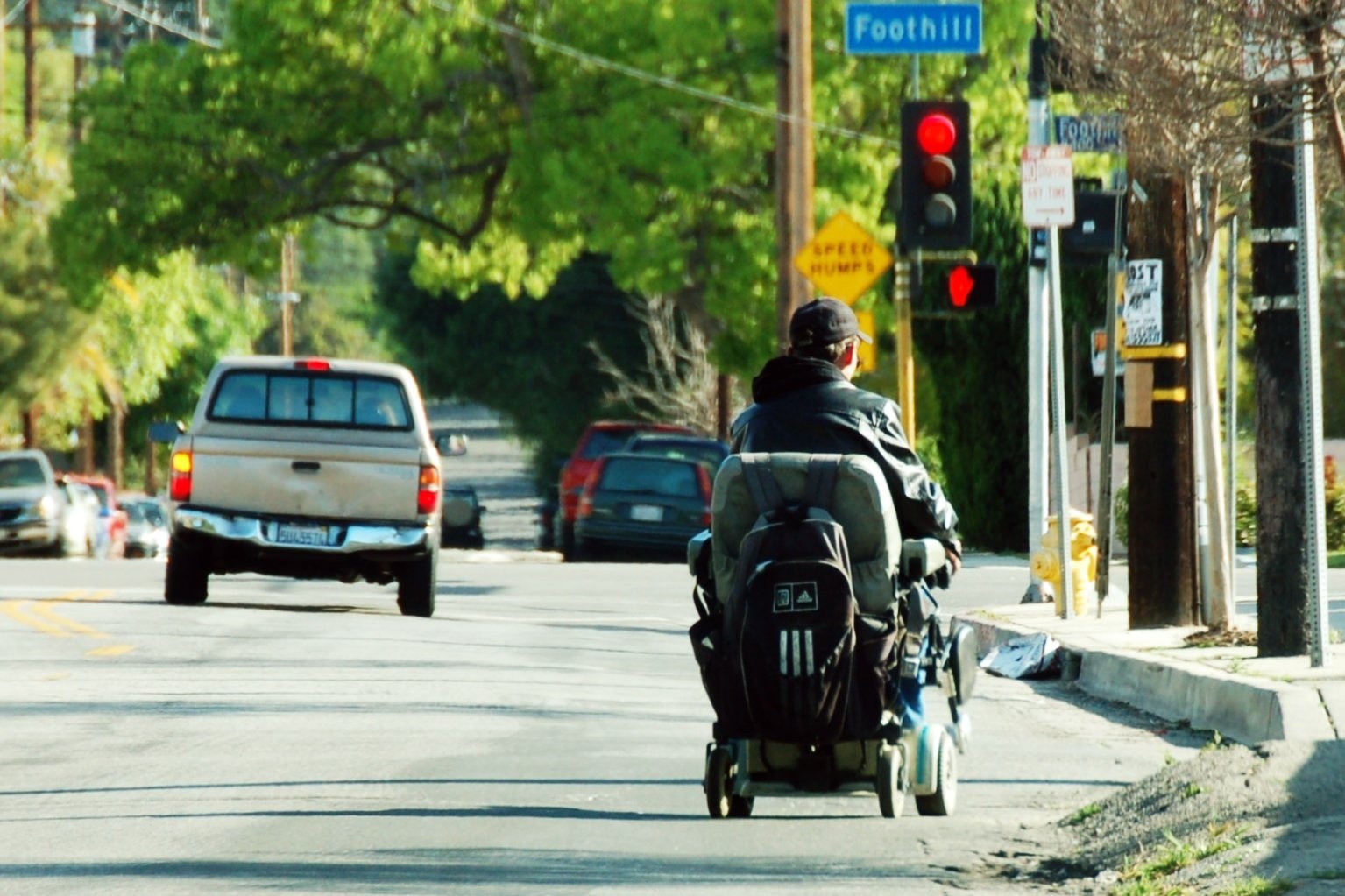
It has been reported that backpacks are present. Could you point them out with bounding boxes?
[717,453,859,752]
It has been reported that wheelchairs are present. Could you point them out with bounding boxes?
[683,451,979,819]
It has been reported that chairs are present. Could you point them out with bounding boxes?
[677,480,695,497]
[356,397,396,423]
[227,385,263,416]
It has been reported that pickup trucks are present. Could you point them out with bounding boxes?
[147,354,471,617]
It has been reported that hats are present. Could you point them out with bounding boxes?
[789,296,873,347]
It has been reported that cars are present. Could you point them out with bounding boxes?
[0,450,171,561]
[442,487,485,552]
[551,420,732,565]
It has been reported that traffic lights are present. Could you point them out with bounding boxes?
[897,98,977,252]
[914,254,983,319]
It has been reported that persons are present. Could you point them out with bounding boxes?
[730,298,962,578]
